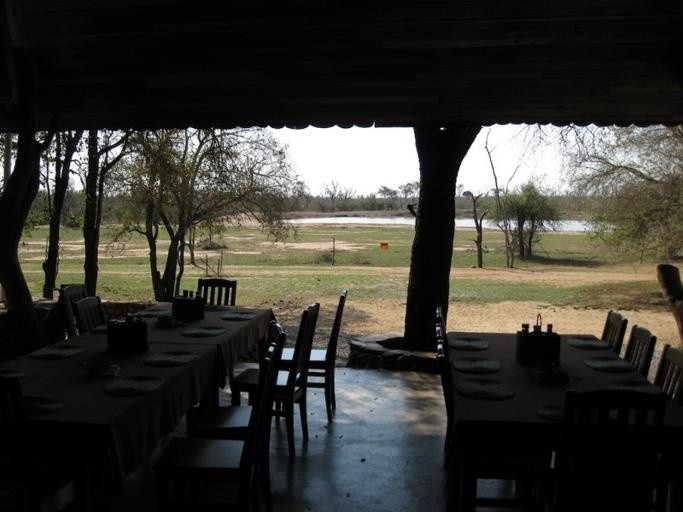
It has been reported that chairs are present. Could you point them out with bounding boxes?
[196,278,237,307]
[230,303,320,456]
[281,290,349,423]
[600,310,628,356]
[623,325,657,379]
[436,308,446,408]
[210,320,287,512]
[652,344,683,400]
[154,342,279,512]
[518,389,671,512]
[56,282,106,339]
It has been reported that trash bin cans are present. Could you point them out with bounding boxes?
[381,242,389,250]
